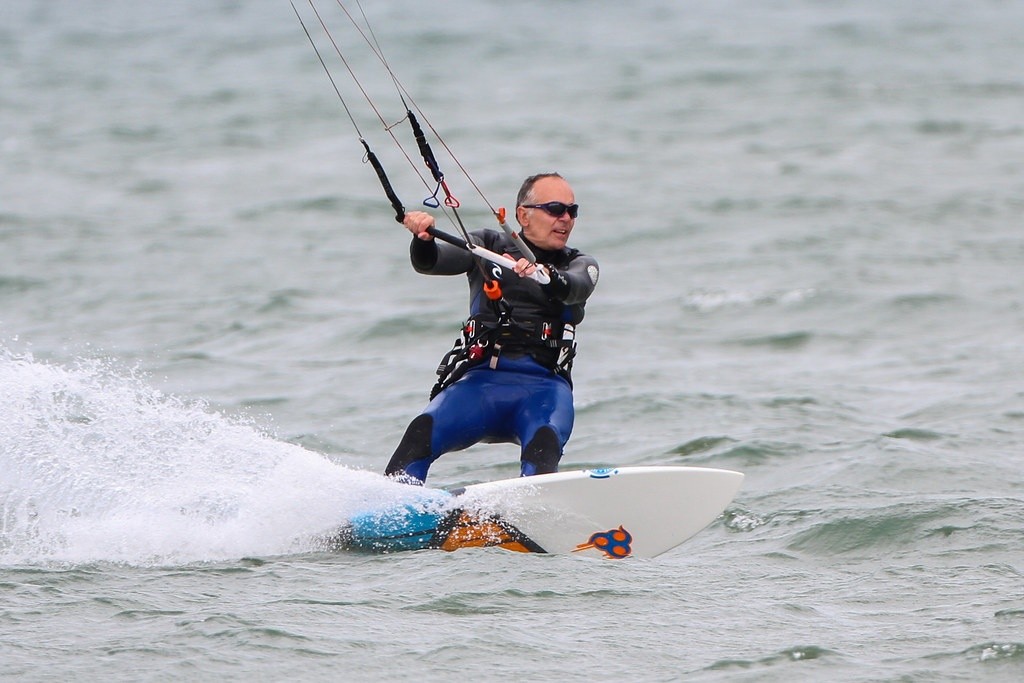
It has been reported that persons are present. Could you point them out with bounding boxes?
[382,171,599,486]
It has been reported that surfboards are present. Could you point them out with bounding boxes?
[292,465,745,562]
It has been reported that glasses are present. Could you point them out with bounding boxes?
[521,202,579,219]
